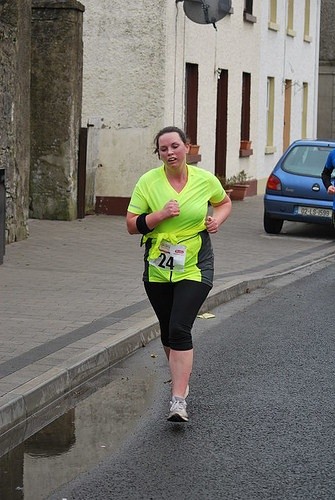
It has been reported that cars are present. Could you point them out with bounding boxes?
[263,138,335,234]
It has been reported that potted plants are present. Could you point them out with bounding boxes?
[229,170,250,201]
[216,173,233,197]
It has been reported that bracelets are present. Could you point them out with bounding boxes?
[136,212,154,235]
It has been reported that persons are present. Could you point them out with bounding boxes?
[320,149,335,228]
[126,127,233,422]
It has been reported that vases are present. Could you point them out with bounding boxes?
[189,145,200,155]
[241,139,252,150]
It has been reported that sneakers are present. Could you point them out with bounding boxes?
[167,395,189,422]
